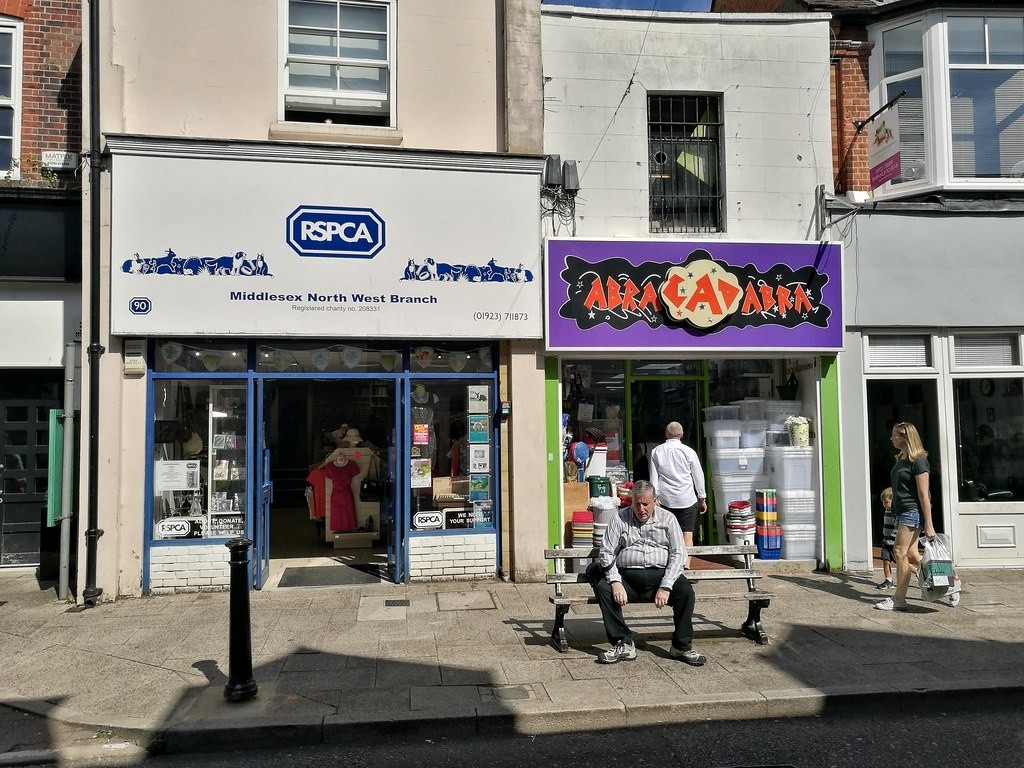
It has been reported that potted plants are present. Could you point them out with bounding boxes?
[563,461,590,548]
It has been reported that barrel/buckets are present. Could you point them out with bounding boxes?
[725,488,782,562]
[571,446,618,573]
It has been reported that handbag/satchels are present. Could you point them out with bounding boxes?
[586,562,606,591]
[359,455,385,503]
[918,533,962,602]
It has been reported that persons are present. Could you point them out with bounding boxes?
[595,479,707,666]
[874,421,961,610]
[632,424,660,480]
[402,385,440,478]
[648,421,708,570]
[876,487,920,589]
[324,448,361,531]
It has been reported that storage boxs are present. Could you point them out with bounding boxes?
[569,419,631,573]
[701,399,820,560]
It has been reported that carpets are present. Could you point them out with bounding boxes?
[278,564,381,587]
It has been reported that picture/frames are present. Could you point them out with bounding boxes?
[986,407,995,422]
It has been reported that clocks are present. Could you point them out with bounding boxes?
[979,379,995,397]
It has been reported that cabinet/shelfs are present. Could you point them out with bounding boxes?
[208,385,248,538]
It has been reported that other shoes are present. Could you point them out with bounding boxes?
[877,578,895,589]
[684,567,699,584]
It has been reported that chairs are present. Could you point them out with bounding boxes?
[960,479,1013,501]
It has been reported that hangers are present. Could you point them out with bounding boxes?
[309,449,357,474]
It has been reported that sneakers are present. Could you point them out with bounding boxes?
[669,643,707,666]
[875,596,908,611]
[949,592,960,607]
[598,639,637,664]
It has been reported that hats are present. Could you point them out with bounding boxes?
[342,429,363,441]
[183,432,203,455]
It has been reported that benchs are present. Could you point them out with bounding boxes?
[545,539,777,653]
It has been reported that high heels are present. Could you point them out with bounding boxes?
[356,514,374,533]
